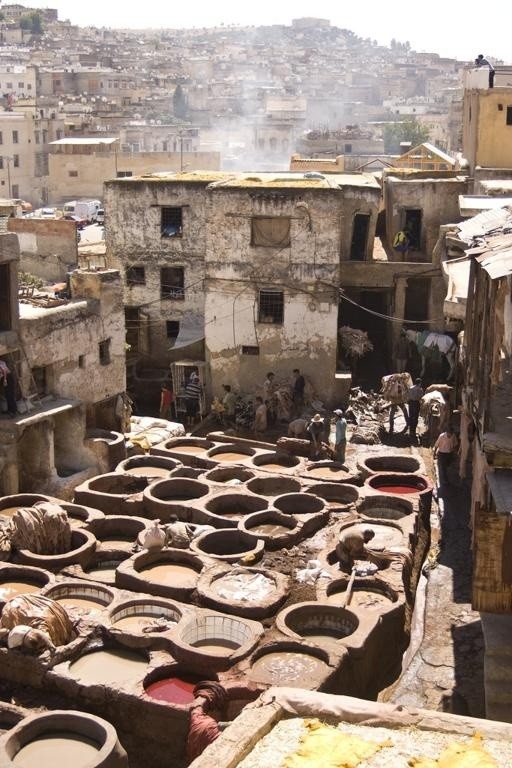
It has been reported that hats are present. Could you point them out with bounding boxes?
[333,409,343,417]
[311,414,324,422]
[415,377,423,383]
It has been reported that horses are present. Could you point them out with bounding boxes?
[401,327,459,381]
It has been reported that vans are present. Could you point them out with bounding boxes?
[40,199,104,229]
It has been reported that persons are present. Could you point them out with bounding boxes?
[0,359,17,419]
[0,625,55,651]
[335,529,375,570]
[160,368,346,464]
[392,227,411,261]
[389,333,476,485]
[164,514,195,550]
[475,54,495,88]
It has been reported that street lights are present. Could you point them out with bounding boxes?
[3,155,15,199]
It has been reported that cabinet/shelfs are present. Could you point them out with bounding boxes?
[171,360,209,422]
